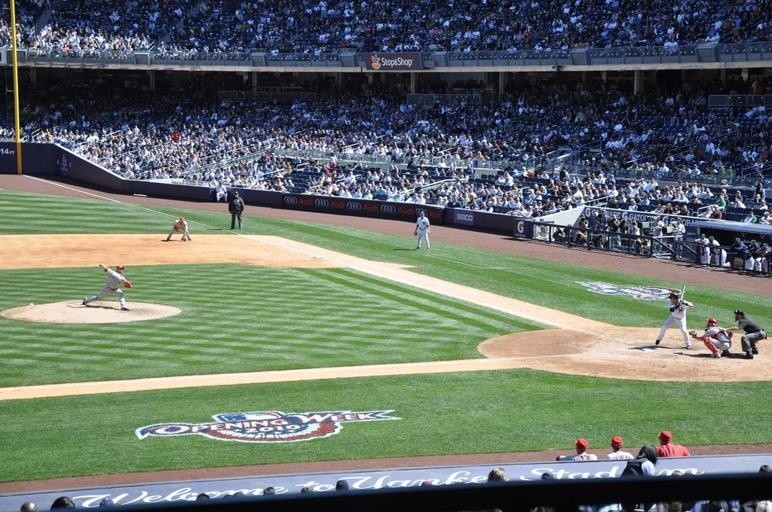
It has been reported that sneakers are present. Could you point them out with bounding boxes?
[742,354,752,359]
[711,352,722,358]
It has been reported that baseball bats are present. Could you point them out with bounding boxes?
[679,285,685,310]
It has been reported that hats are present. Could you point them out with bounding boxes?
[575,438,588,451]
[610,436,623,449]
[658,431,672,443]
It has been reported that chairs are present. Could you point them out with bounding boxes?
[1,85,771,226]
[0,1,772,60]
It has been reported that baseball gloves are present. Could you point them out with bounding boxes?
[689,331,697,338]
[124,281,131,289]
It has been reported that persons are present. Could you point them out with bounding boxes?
[733,307,768,358]
[82,263,133,311]
[519,0,771,281]
[50,494,77,511]
[215,180,228,201]
[196,492,209,501]
[262,485,275,495]
[539,429,690,478]
[686,315,733,359]
[413,212,430,250]
[486,467,506,481]
[758,463,771,473]
[20,501,37,511]
[167,217,191,241]
[655,287,694,349]
[233,0,524,215]
[0,1,237,190]
[336,480,350,490]
[229,191,245,230]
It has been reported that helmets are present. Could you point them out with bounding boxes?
[668,292,679,299]
[734,310,745,322]
[705,316,716,328]
